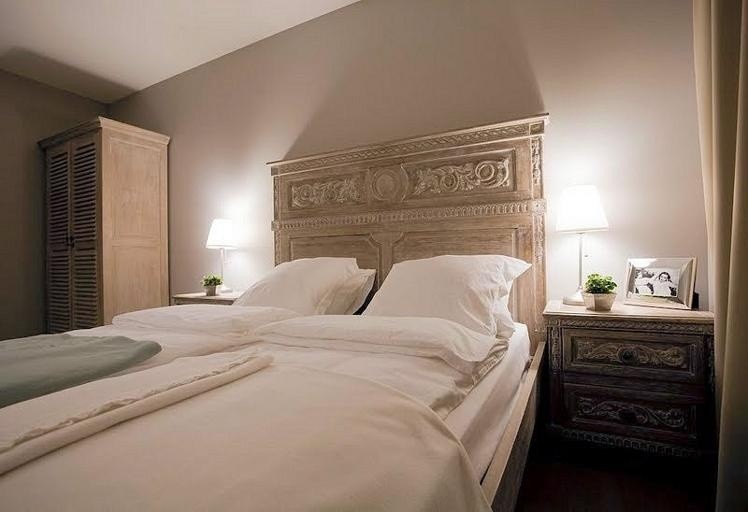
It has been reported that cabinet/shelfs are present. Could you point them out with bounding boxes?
[36,117,172,331]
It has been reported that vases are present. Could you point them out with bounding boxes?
[200,272,223,296]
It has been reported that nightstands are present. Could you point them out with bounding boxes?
[173,292,239,305]
[542,300,713,466]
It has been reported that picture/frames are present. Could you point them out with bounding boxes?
[623,257,697,311]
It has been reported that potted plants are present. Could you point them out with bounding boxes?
[581,273,617,311]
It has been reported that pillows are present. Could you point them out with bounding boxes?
[361,255,532,340]
[231,256,377,315]
[327,269,375,313]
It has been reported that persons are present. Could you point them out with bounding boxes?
[633,270,652,295]
[647,271,677,297]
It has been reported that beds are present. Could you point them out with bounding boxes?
[0,111,549,512]
